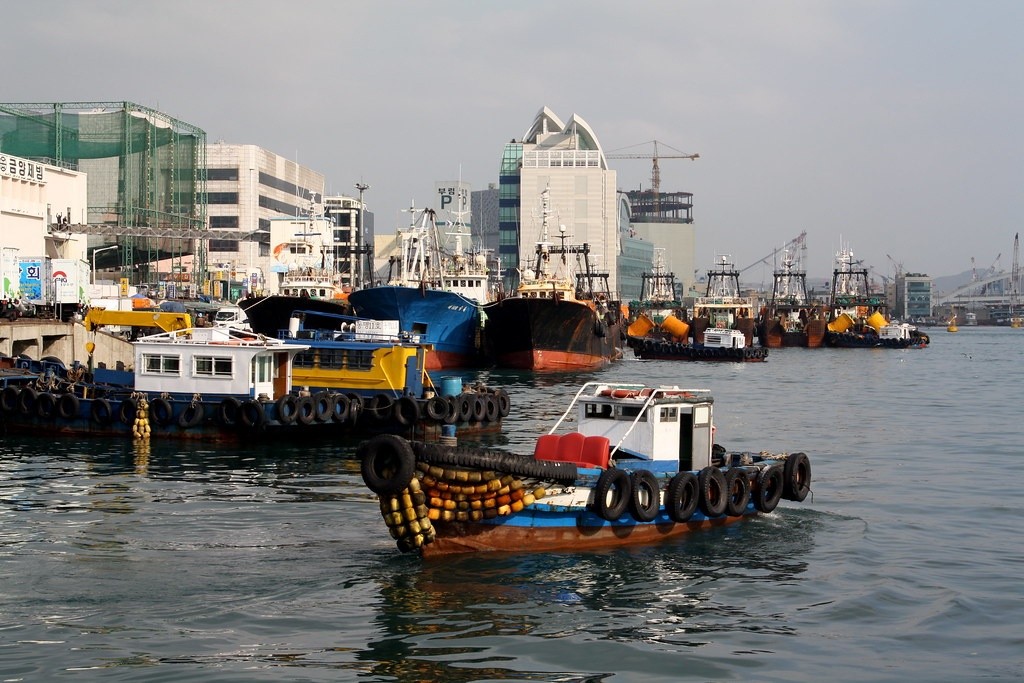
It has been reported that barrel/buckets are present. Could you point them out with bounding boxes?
[288,317,300,339]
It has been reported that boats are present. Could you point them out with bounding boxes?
[480,177,612,375]
[623,303,769,363]
[363,384,815,563]
[234,184,355,342]
[693,255,755,346]
[826,249,931,348]
[348,206,480,370]
[0,316,512,441]
[760,243,826,349]
[623,245,686,333]
[211,305,254,335]
[418,162,490,307]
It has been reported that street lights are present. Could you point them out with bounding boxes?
[93,245,118,286]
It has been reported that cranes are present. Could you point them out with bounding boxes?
[601,139,702,218]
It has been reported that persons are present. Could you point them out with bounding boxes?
[193,312,210,328]
[6,296,21,323]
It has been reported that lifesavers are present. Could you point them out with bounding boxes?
[600,387,692,397]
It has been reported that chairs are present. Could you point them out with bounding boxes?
[575,435,610,469]
[554,432,585,464]
[533,435,560,461]
